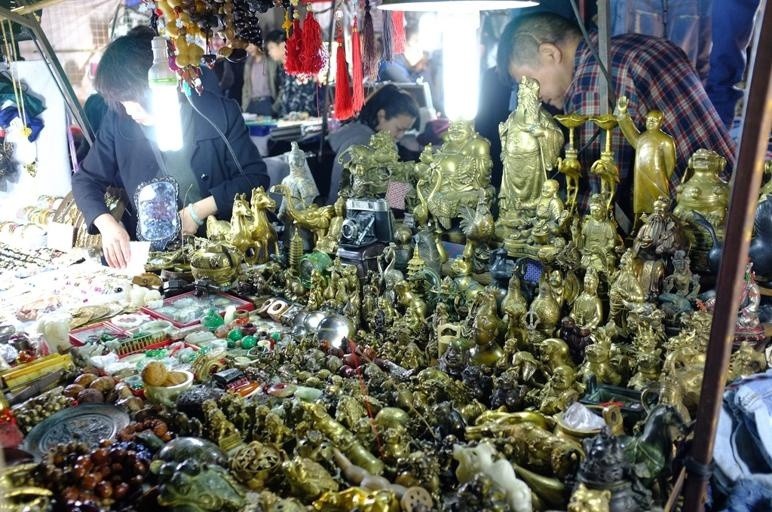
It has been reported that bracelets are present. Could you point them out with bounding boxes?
[187,202,203,228]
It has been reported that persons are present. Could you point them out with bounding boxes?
[328,83,422,205]
[65,44,100,89]
[266,27,323,118]
[633,196,683,302]
[694,255,766,351]
[545,270,564,309]
[316,195,346,253]
[212,36,247,109]
[75,92,96,163]
[616,95,678,236]
[662,250,700,300]
[377,28,419,86]
[571,193,617,274]
[569,266,602,337]
[657,378,693,429]
[608,246,644,322]
[496,10,739,218]
[242,39,276,116]
[516,178,568,236]
[413,120,495,216]
[70,25,271,270]
[495,75,564,243]
[538,366,578,416]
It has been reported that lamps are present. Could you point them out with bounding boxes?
[147,37,183,152]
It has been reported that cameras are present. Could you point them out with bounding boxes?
[340,198,393,250]
